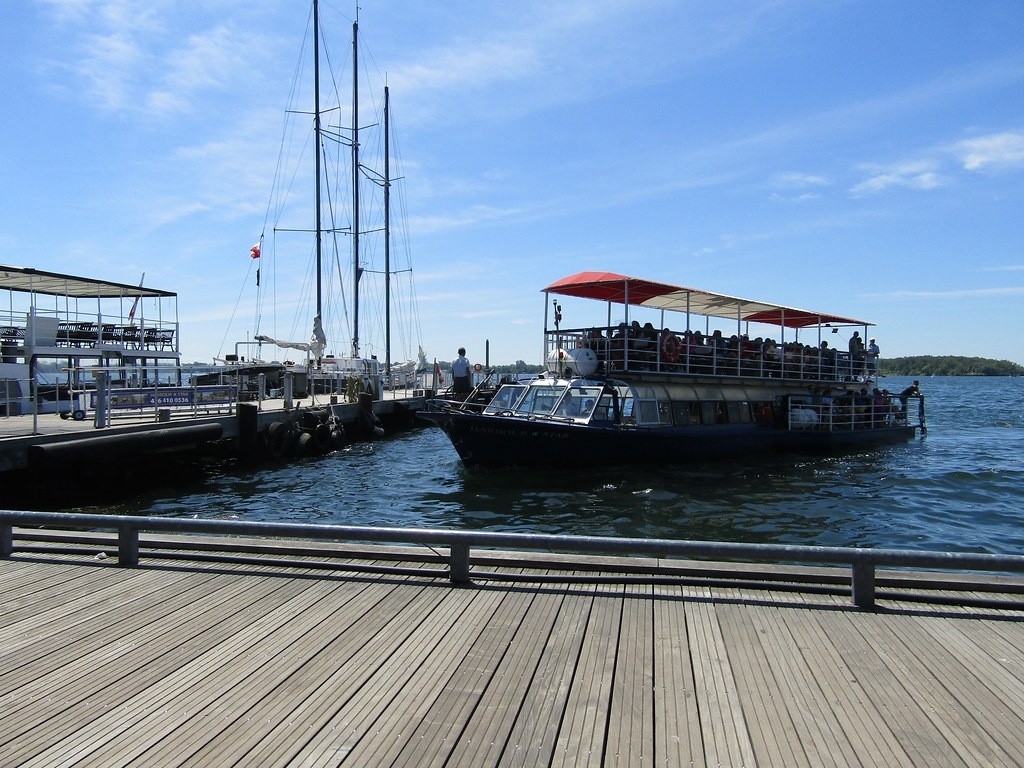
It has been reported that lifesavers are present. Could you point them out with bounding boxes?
[661,332,681,362]
[264,417,346,456]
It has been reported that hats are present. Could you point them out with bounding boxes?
[913,381,919,384]
[743,334,749,338]
[870,338,875,342]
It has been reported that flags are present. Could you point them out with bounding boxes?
[257,269,259,286]
[129,282,142,319]
[435,363,444,384]
[250,241,260,259]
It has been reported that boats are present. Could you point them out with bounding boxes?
[415,271,929,486]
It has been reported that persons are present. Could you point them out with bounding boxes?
[582,321,738,377]
[805,385,899,430]
[450,347,473,402]
[900,381,920,418]
[847,331,880,383]
[740,336,844,381]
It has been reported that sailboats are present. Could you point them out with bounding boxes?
[0,8,422,415]
